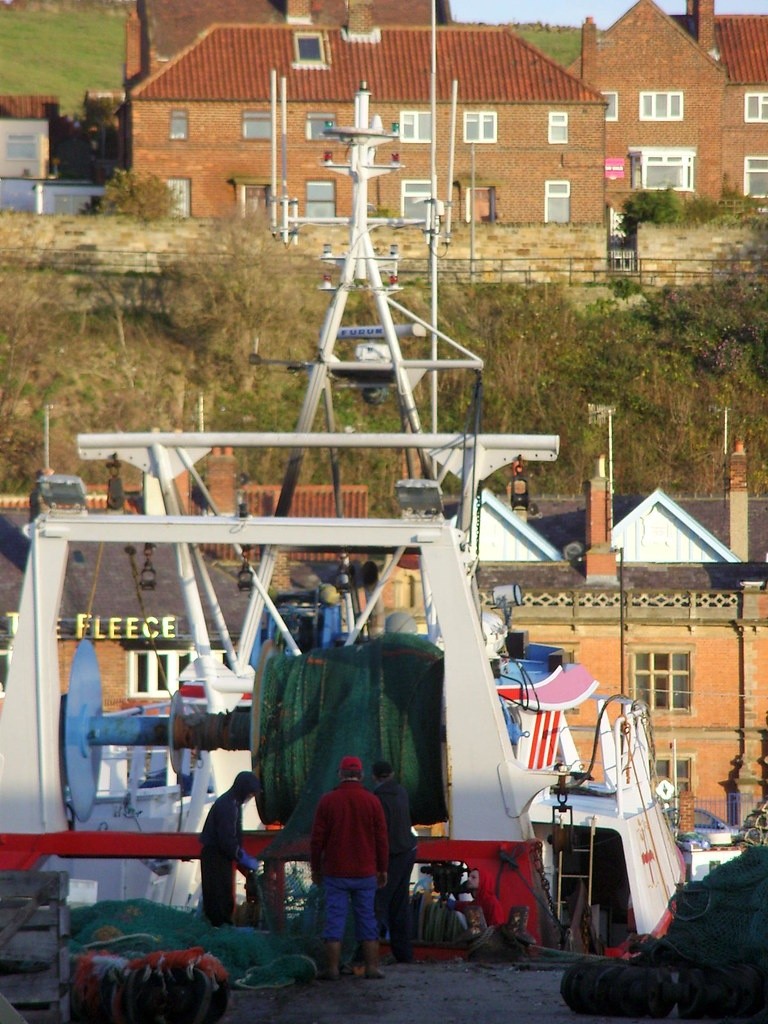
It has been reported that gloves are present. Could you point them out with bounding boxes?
[238,849,258,871]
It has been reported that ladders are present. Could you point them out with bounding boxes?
[556,816,598,920]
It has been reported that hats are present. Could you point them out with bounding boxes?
[339,756,362,770]
[372,762,392,777]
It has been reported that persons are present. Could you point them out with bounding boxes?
[198,771,257,927]
[309,755,389,983]
[454,866,505,927]
[371,759,417,966]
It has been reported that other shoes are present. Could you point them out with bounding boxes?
[378,951,398,964]
[392,947,411,964]
[316,967,339,981]
[365,965,386,978]
